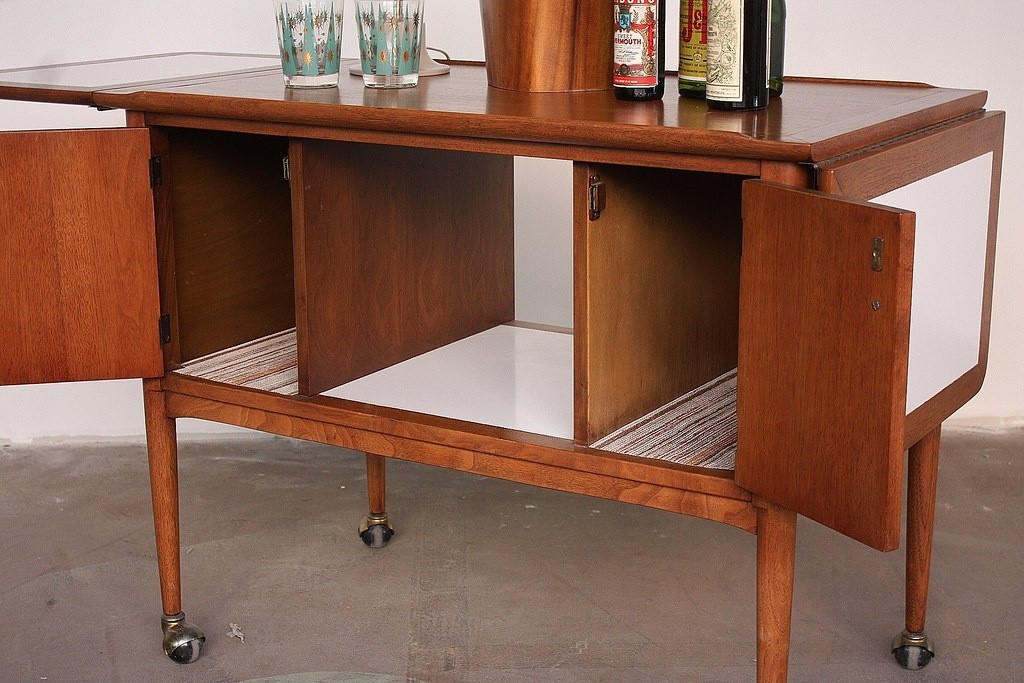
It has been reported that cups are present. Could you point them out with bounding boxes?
[355,0,424,89]
[272,0,345,89]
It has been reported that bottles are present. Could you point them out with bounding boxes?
[612,0,665,101]
[678,0,786,112]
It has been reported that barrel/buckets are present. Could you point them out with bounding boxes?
[480,0,612,94]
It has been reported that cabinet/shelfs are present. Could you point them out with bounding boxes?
[0,49,1007,682]
